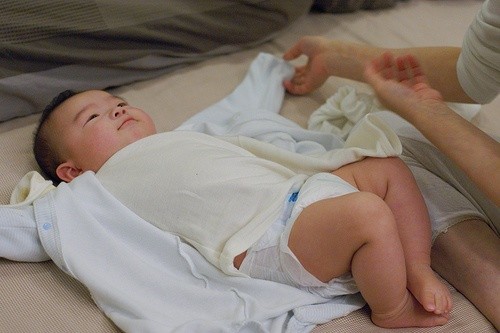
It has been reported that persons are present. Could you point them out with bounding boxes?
[0,53,453,333]
[280,0,500,333]
